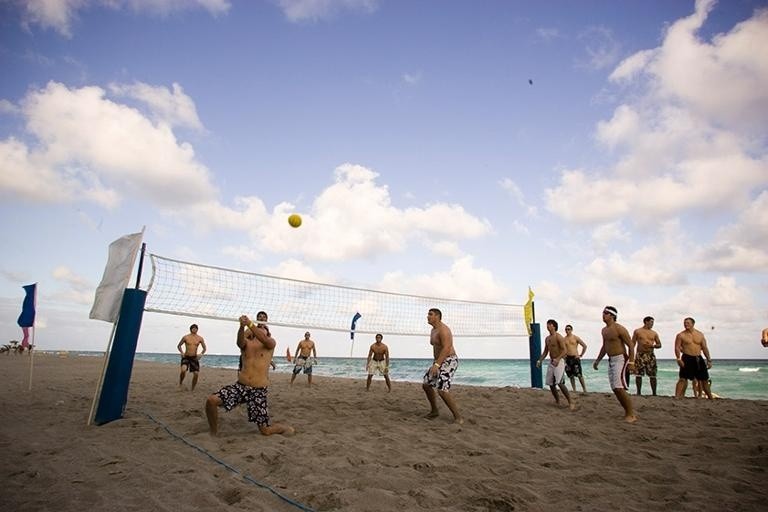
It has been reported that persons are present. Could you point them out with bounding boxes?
[176,322,207,392]
[534,306,723,421]
[204,315,294,439]
[761,327,767,347]
[290,331,318,389]
[365,333,392,393]
[420,308,465,426]
[238,311,275,379]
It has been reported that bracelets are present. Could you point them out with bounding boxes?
[434,363,441,367]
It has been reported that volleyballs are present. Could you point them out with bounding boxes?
[288,215,302,227]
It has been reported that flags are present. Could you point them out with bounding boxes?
[16,283,36,328]
[523,289,534,336]
[286,347,292,363]
[88,232,141,324]
[349,313,362,340]
